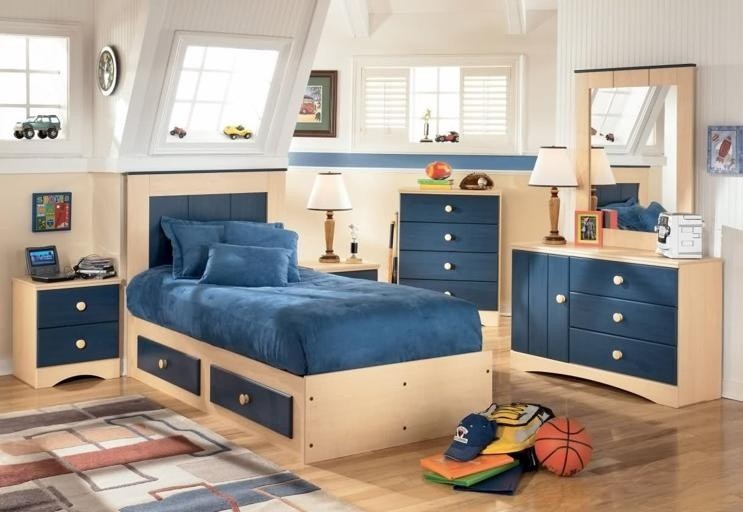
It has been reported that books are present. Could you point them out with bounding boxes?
[420,185,452,190]
[418,179,453,184]
[453,466,522,495]
[425,459,521,486]
[420,455,514,481]
[76,259,115,279]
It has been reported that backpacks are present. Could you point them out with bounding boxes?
[480,402,555,473]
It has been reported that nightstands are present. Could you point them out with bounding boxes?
[299,261,381,283]
[11,275,124,389]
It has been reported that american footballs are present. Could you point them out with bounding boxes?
[425,161,453,180]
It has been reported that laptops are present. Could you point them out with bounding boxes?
[26,245,75,281]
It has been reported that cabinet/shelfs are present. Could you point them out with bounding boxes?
[397,189,503,329]
[506,243,727,409]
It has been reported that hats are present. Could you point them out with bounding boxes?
[443,414,496,463]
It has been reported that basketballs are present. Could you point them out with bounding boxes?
[535,417,593,477]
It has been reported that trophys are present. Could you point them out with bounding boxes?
[420,109,432,141]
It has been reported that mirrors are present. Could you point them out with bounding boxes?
[572,63,699,254]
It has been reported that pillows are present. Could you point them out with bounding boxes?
[160,215,286,281]
[597,194,638,211]
[197,241,294,287]
[606,202,646,229]
[222,220,301,283]
[640,201,668,231]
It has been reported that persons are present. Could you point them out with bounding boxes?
[581,218,594,240]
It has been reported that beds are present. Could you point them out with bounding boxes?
[121,168,495,464]
[593,162,664,231]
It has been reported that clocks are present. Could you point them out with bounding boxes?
[94,44,119,97]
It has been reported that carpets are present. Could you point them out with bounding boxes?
[0,391,348,512]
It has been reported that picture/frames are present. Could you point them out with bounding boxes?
[706,124,743,177]
[291,69,340,139]
[573,210,606,249]
[31,192,72,232]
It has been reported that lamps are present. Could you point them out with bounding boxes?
[306,170,354,264]
[591,145,617,211]
[526,143,581,245]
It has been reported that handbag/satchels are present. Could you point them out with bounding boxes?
[478,402,556,454]
